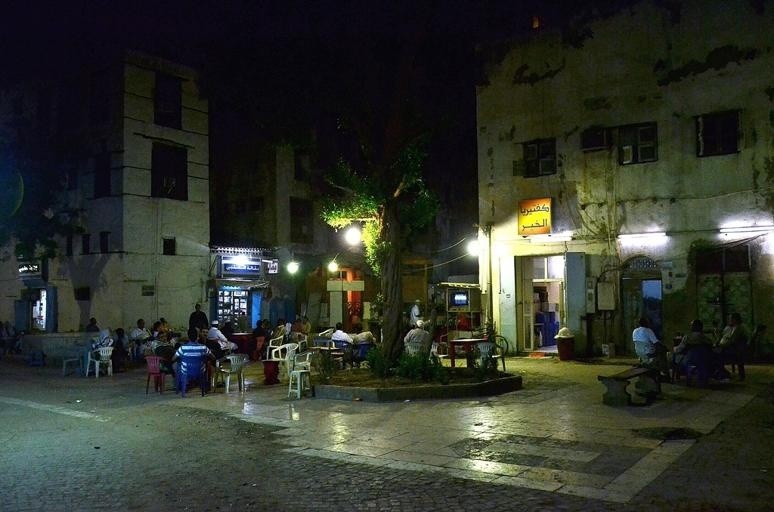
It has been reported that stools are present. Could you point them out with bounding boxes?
[29,349,44,367]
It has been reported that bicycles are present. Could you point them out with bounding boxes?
[453,316,508,357]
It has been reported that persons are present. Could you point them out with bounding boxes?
[403,320,429,356]
[409,299,424,327]
[247,316,311,360]
[188,303,208,332]
[160,317,168,331]
[145,321,164,351]
[128,318,151,353]
[352,323,372,353]
[331,321,353,351]
[152,331,177,355]
[171,328,216,392]
[633,315,681,384]
[714,312,748,382]
[85,318,100,332]
[109,327,129,374]
[198,328,219,366]
[206,320,238,358]
[672,319,712,370]
[220,318,236,340]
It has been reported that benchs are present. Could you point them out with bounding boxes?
[597,362,662,406]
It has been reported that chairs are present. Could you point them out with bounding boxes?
[632,329,748,385]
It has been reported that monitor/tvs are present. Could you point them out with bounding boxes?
[451,292,468,305]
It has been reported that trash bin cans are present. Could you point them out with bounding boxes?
[554,327,575,360]
